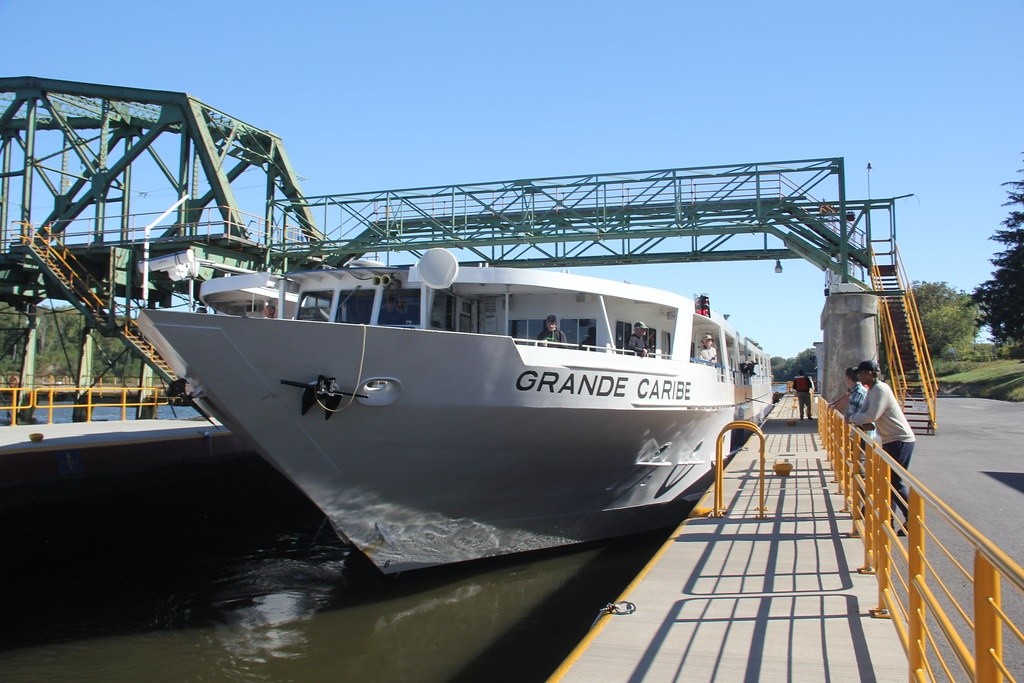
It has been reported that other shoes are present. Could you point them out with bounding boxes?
[800,417,803,420]
[891,519,895,529]
[897,521,909,536]
[808,417,813,420]
[859,506,866,519]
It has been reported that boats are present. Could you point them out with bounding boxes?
[136,247,773,577]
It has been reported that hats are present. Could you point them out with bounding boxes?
[702,334,715,342]
[634,321,648,329]
[852,360,879,373]
[547,315,556,323]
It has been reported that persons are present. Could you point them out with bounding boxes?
[579,327,596,351]
[792,370,813,420]
[264,301,276,318]
[697,335,717,366]
[845,360,916,536]
[628,321,654,357]
[537,315,568,348]
[826,366,876,519]
